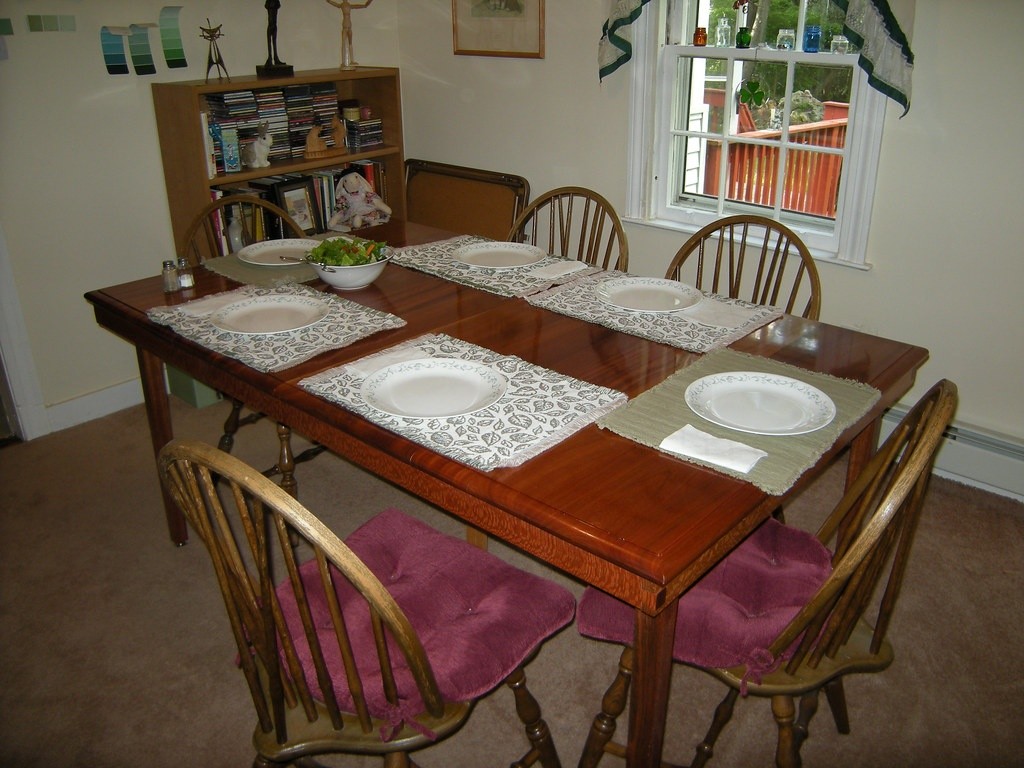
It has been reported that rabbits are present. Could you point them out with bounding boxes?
[240,120,273,168]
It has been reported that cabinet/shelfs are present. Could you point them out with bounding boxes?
[151,65,407,267]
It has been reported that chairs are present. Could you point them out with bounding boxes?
[507,186,629,275]
[155,441,576,768]
[663,212,820,321]
[577,379,959,768]
[182,195,328,545]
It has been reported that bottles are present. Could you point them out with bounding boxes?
[735,26,752,47]
[161,260,181,294]
[176,256,195,288]
[802,25,821,53]
[776,28,795,51]
[829,35,848,56]
[693,27,707,46]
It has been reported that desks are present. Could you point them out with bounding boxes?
[84,217,929,768]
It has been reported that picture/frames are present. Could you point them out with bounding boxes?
[273,175,323,238]
[450,0,545,59]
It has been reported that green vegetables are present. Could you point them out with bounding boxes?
[304,237,388,266]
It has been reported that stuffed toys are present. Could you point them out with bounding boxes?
[328,172,392,228]
[241,120,273,169]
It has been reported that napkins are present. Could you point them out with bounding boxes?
[180,292,251,319]
[676,297,756,329]
[345,347,431,378]
[527,260,589,280]
[660,424,769,473]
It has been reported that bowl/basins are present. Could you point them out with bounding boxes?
[307,246,395,291]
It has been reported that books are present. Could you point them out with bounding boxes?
[210,159,388,258]
[208,91,383,171]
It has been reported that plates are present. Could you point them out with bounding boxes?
[209,295,329,335]
[594,276,704,314]
[360,357,507,418]
[237,238,323,265]
[684,371,837,437]
[451,242,546,271]
